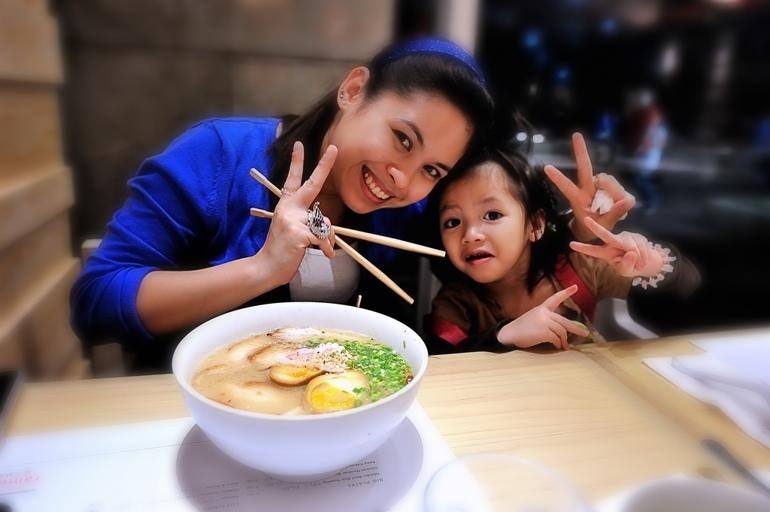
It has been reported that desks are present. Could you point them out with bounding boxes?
[0,315,770,511]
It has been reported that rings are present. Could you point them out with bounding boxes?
[306,200,331,238]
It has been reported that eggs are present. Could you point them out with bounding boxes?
[268,365,370,413]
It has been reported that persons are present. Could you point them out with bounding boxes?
[423,154,702,353]
[69,38,638,377]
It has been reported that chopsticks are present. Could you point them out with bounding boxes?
[245,166,445,303]
[171,301,429,484]
